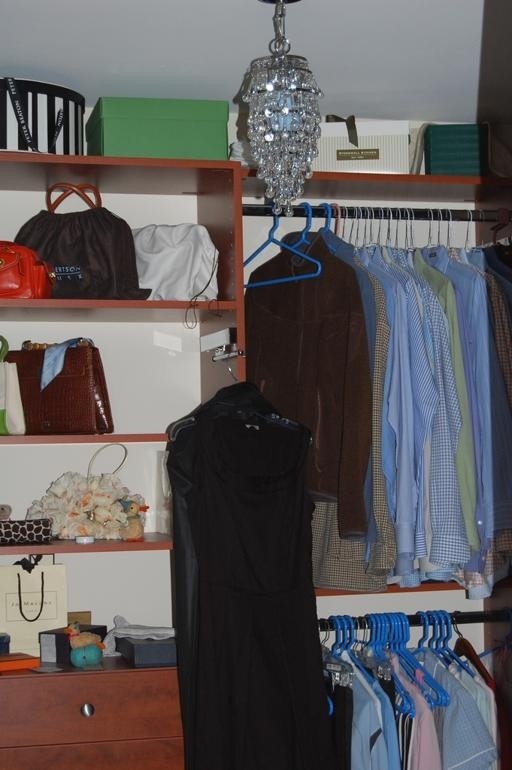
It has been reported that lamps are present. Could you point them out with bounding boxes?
[243,0,326,217]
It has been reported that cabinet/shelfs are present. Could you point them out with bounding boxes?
[0,150,243,770]
[246,173,512,770]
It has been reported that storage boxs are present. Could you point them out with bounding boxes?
[311,120,488,175]
[0,77,229,161]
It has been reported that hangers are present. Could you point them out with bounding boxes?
[320,609,497,718]
[243,201,512,291]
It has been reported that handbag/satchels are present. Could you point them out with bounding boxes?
[1,563,69,658]
[0,240,57,301]
[131,221,221,330]
[23,443,151,543]
[13,181,140,300]
[0,337,114,435]
[0,75,87,157]
[0,518,54,547]
